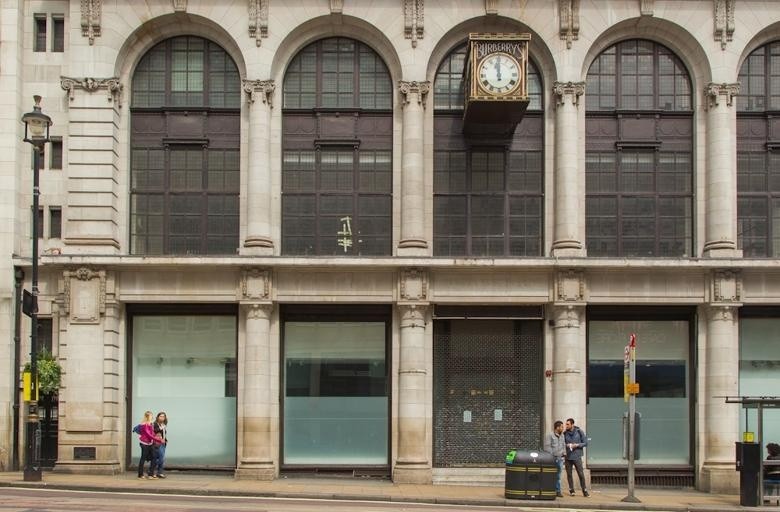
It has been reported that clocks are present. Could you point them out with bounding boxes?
[468,32,531,103]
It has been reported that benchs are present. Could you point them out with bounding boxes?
[763,479,780,506]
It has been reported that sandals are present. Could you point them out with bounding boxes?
[139,470,166,480]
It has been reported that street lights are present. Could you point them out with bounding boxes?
[21,93,53,482]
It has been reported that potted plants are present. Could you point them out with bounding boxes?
[22,348,63,471]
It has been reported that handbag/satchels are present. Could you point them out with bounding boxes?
[133,424,141,434]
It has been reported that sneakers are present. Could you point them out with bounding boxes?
[569,488,576,496]
[582,487,589,497]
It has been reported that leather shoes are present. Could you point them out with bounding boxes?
[556,492,563,497]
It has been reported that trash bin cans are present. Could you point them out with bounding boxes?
[504,450,558,500]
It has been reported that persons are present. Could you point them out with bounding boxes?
[137,411,165,480]
[545,421,567,499]
[764,443,780,503]
[563,418,592,497]
[153,412,168,478]
